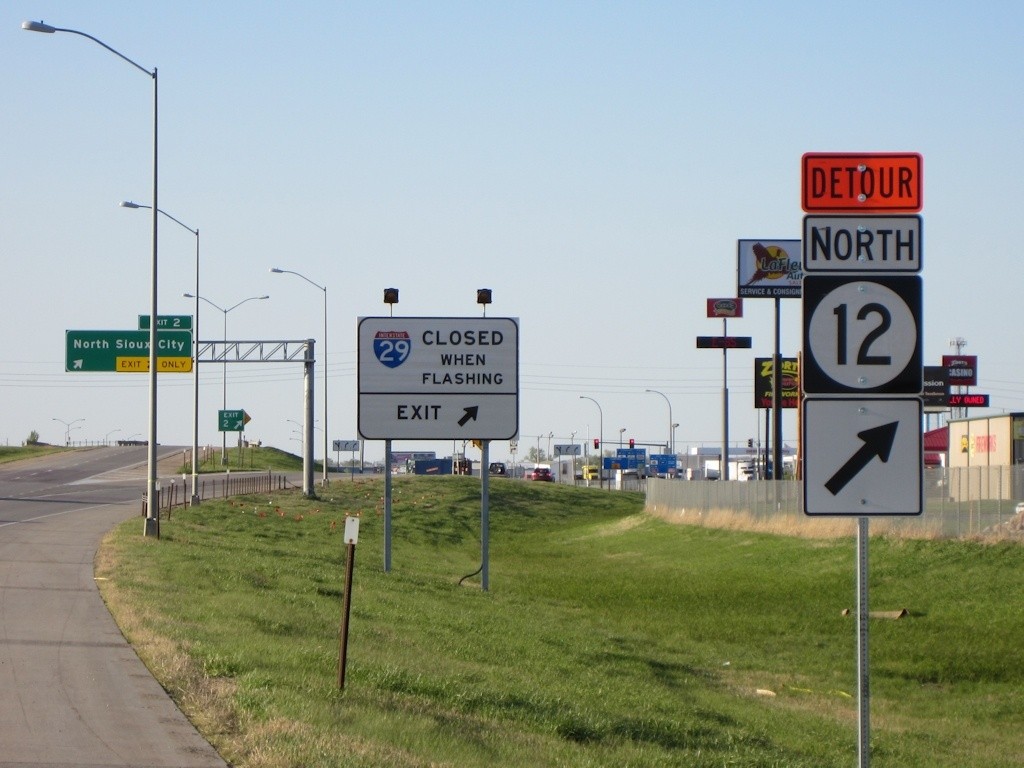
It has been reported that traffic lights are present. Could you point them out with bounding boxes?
[628,438,636,450]
[594,438,599,449]
[747,439,753,448]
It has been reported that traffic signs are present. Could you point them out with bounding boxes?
[65,330,194,375]
[216,409,245,433]
[354,313,521,442]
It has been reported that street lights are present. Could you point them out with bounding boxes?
[127,434,143,441]
[269,268,332,487]
[18,19,163,539]
[64,426,83,445]
[579,395,604,489]
[118,199,200,506]
[182,292,270,466]
[105,429,121,447]
[536,434,545,466]
[643,388,675,455]
[570,430,577,444]
[946,334,967,420]
[51,418,86,445]
[286,419,320,452]
[547,432,554,462]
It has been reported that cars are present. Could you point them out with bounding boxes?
[531,467,556,483]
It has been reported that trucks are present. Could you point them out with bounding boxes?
[581,465,600,481]
